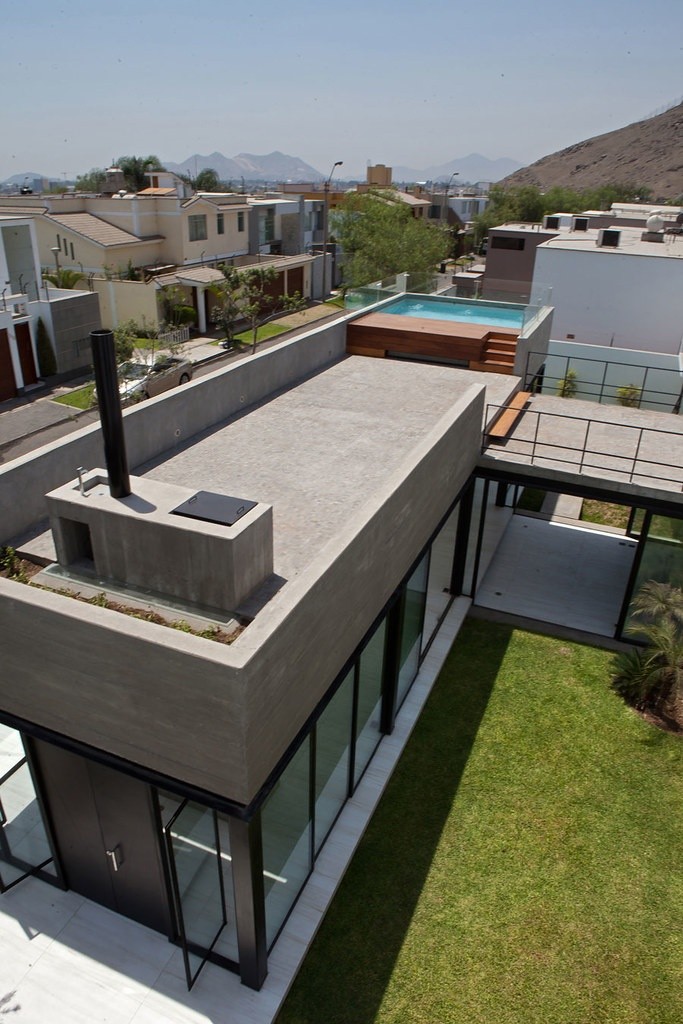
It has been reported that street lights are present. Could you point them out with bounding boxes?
[322,161,343,303]
[444,173,459,222]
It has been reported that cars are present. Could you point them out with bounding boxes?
[479,237,488,252]
[92,351,193,409]
